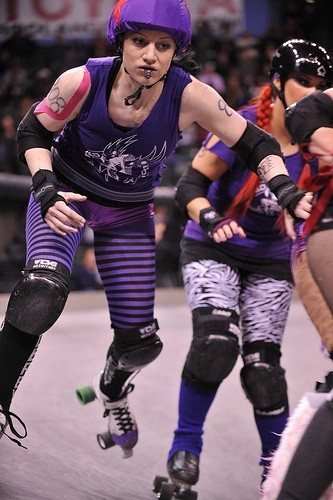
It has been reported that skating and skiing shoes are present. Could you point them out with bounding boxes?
[0,404,27,451]
[152,450,199,500]
[75,372,139,458]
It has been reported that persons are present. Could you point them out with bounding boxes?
[260,88,332,500]
[152,39,333,500]
[0,0,313,460]
[0,0,333,171]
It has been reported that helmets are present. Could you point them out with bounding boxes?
[269,39,333,79]
[108,0,191,56]
